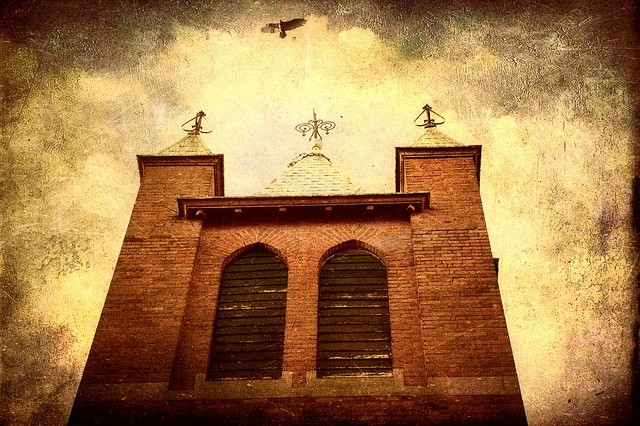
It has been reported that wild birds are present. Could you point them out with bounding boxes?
[260,17,308,39]
